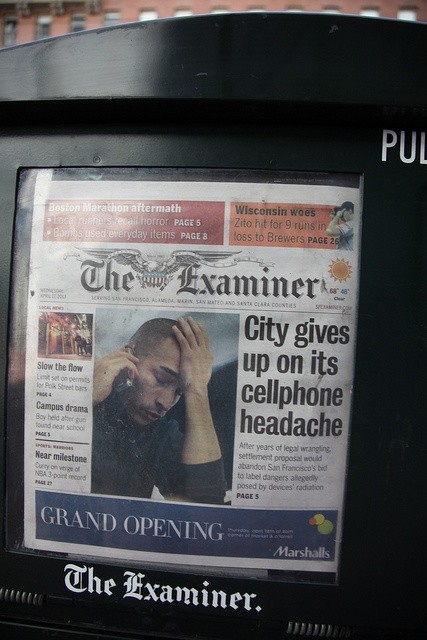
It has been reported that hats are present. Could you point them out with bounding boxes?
[333,202,354,211]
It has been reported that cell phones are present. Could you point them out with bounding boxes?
[115,347,133,393]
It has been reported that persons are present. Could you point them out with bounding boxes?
[326,201,354,250]
[80,337,87,354]
[74,332,84,355]
[91,316,226,508]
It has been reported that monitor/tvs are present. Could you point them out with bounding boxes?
[0,13,427,640]
